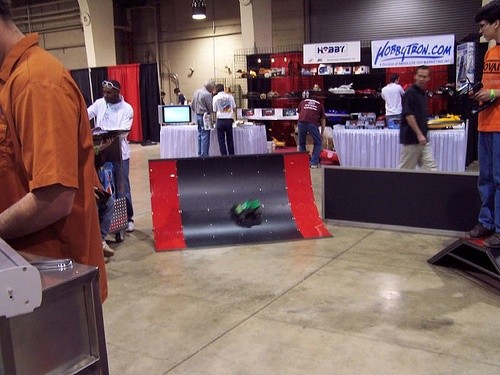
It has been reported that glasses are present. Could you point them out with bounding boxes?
[102,81,120,90]
[478,23,487,29]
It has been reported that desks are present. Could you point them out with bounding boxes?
[333,126,469,173]
[0,249,110,375]
[160,123,268,157]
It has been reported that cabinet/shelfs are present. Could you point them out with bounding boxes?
[241,60,301,109]
[296,59,386,125]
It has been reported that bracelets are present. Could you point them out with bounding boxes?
[489,88,495,99]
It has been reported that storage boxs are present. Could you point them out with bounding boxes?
[300,62,371,75]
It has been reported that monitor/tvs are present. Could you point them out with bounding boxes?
[163,106,191,123]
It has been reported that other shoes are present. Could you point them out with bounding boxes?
[102,240,115,263]
[465,227,495,239]
[310,163,321,169]
[484,235,500,246]
[125,222,136,233]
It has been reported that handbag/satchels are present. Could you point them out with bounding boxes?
[203,112,213,131]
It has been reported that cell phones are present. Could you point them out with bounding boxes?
[28,258,72,270]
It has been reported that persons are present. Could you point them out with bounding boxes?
[87,80,135,233]
[173,88,186,105]
[94,192,115,255]
[398,65,439,172]
[382,74,406,128]
[0,0,109,304]
[449,0,500,247]
[295,96,328,168]
[213,83,237,156]
[190,80,214,156]
[161,92,167,105]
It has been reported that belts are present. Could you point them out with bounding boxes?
[196,114,204,117]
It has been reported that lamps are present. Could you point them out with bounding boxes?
[192,0,208,20]
